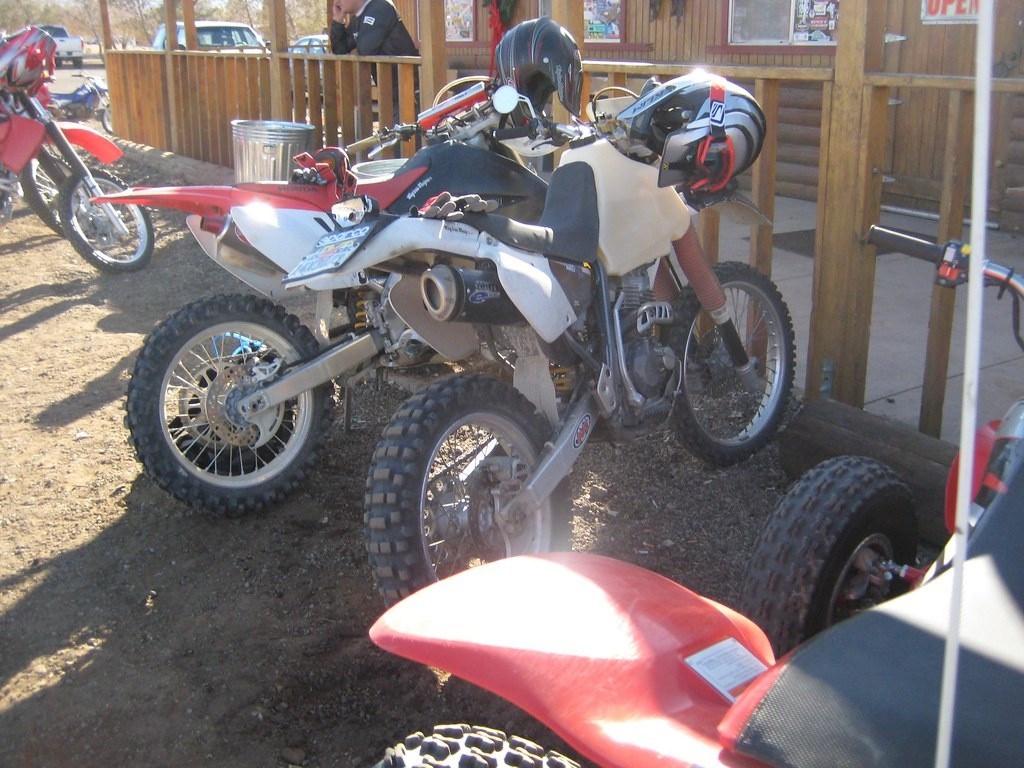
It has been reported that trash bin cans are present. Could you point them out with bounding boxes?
[231,119,316,183]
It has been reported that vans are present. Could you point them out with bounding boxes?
[151,20,274,61]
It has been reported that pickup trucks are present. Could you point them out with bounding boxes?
[22,26,86,76]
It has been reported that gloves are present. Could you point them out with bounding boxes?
[410,192,499,220]
[290,163,337,185]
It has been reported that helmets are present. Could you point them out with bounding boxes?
[0,27,57,93]
[617,71,767,189]
[496,16,584,126]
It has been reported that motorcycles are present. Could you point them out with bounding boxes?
[351,219,1024,768]
[42,73,116,134]
[93,25,577,521]
[278,66,798,633]
[0,26,155,273]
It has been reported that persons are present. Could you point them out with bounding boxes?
[330,0,422,158]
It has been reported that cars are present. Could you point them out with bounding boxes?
[287,32,355,100]
